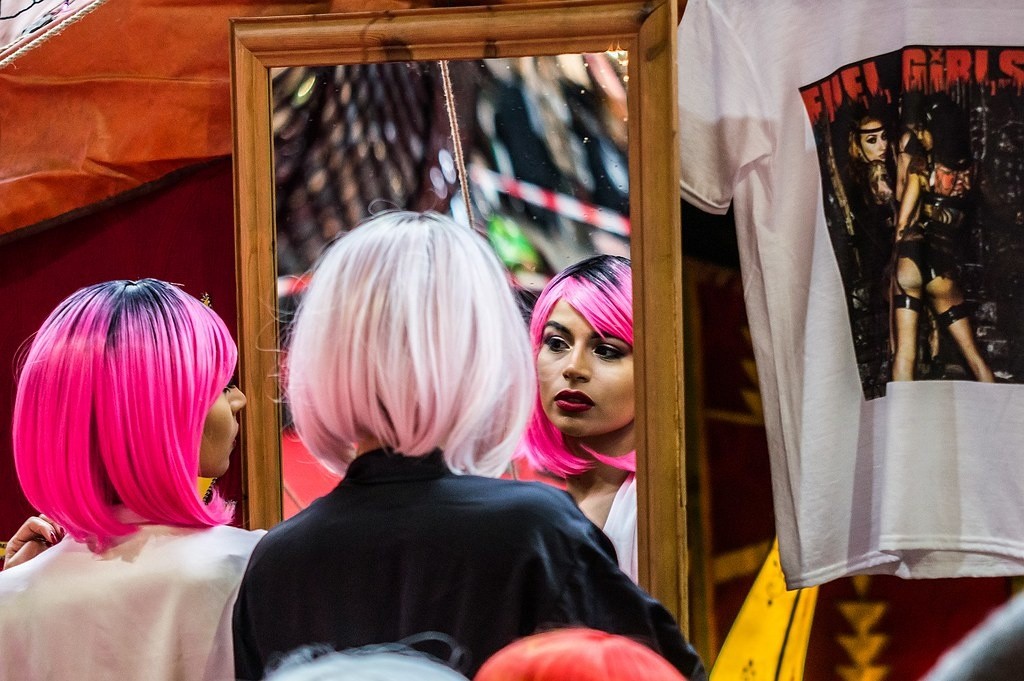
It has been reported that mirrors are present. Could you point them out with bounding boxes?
[232,2,691,655]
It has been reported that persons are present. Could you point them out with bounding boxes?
[230,211,708,681]
[894,93,941,380]
[473,627,684,681]
[892,100,997,382]
[266,638,470,681]
[844,115,944,396]
[522,254,638,588]
[1,280,268,681]
